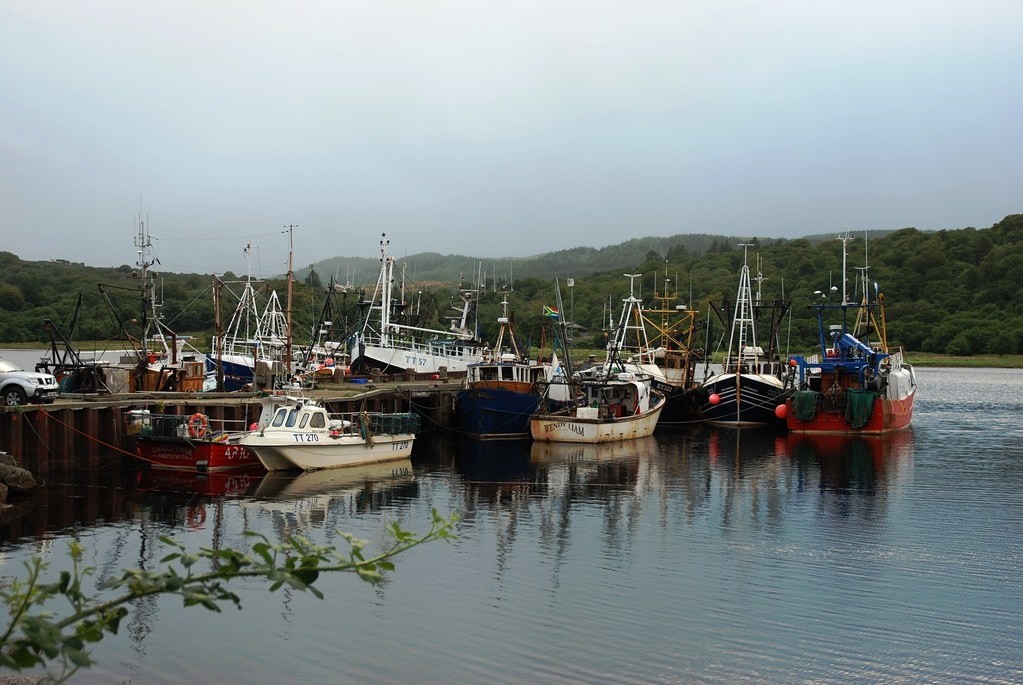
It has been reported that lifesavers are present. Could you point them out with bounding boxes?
[188,413,207,437]
[189,506,206,528]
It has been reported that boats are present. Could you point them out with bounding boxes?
[233,322,421,471]
[33,205,799,444]
[122,404,261,474]
[784,227,919,437]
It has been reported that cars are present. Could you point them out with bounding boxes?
[0,353,61,407]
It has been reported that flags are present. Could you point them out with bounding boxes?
[543,305,561,319]
